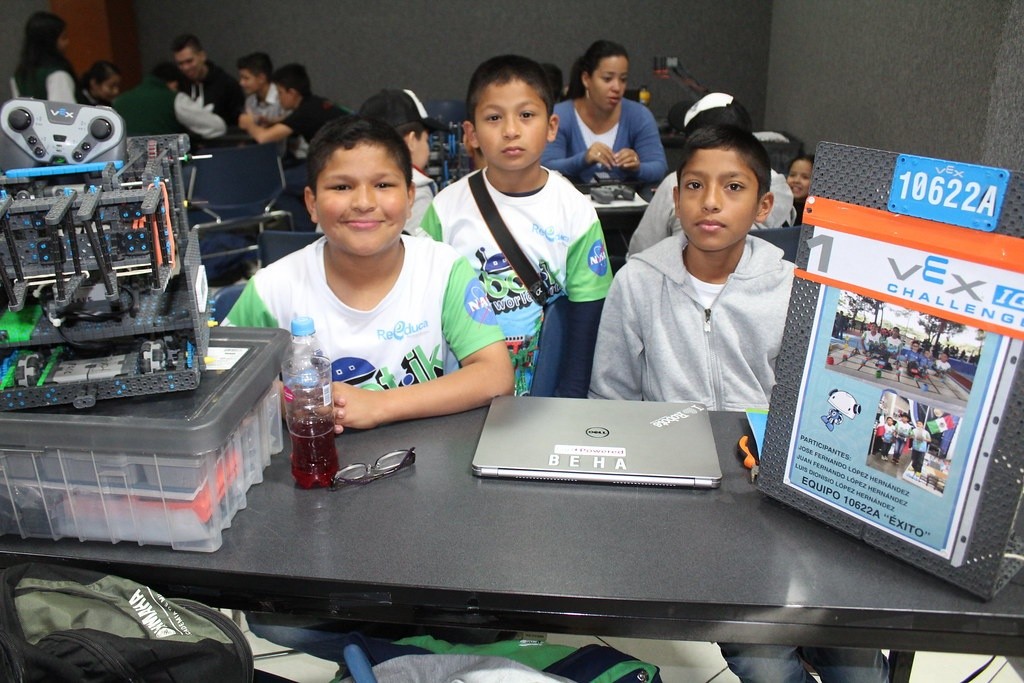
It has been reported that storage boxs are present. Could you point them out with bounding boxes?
[0,326,290,553]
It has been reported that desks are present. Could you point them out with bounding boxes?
[0,412,1024,683]
[577,183,656,255]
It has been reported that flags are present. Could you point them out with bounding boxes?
[927,415,955,434]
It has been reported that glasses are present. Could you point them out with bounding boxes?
[333,447,416,483]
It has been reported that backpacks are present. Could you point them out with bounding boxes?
[0,562,254,683]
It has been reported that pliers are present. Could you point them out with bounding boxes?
[737,435,759,482]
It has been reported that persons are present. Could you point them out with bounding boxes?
[832,310,981,378]
[780,155,814,228]
[220,114,515,435]
[237,53,309,170]
[113,61,226,139]
[239,63,347,145]
[882,412,931,476]
[541,40,668,184]
[588,123,796,412]
[314,90,450,237]
[464,113,488,169]
[9,11,79,103]
[172,34,246,127]
[414,55,614,399]
[626,92,794,266]
[79,60,124,106]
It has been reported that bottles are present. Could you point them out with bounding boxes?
[280,317,340,489]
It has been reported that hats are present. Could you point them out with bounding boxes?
[358,88,443,139]
[678,91,751,138]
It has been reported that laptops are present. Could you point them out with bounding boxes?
[472,396,723,489]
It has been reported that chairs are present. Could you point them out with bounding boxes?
[167,150,320,320]
[418,98,473,192]
